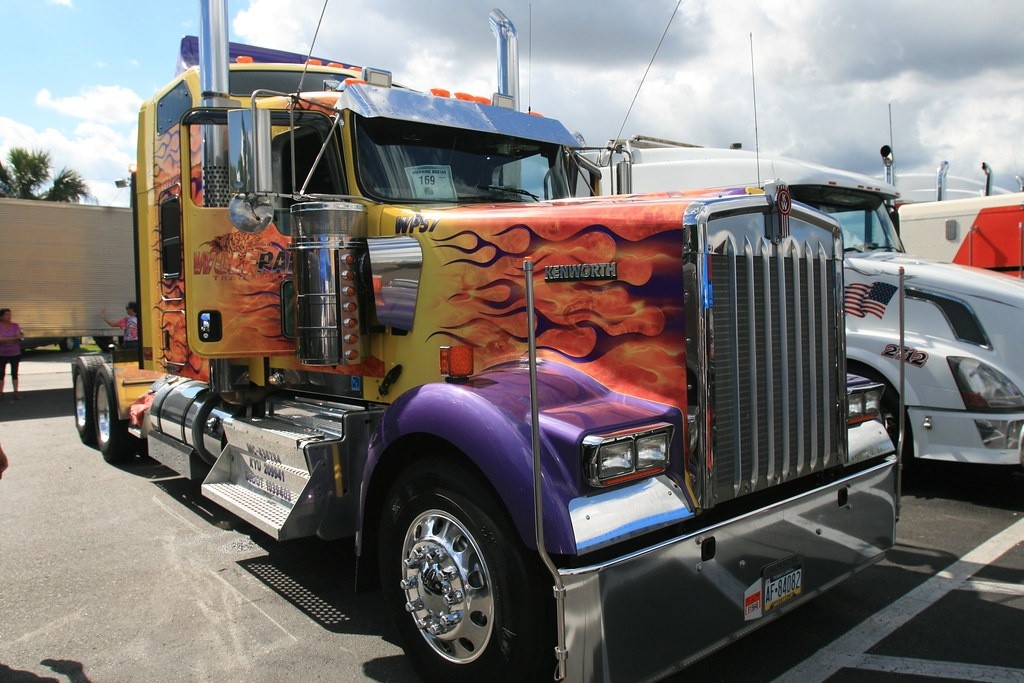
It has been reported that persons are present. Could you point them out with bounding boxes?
[101,302,138,350]
[0,308,25,400]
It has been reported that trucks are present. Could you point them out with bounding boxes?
[494,135,1024,492]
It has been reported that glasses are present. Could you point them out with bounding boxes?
[126,306,128,309]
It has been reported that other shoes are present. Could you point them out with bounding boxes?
[12,393,23,401]
[0,392,4,399]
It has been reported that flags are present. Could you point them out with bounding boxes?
[842,281,899,318]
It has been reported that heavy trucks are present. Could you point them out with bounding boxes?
[68,0,907,683]
